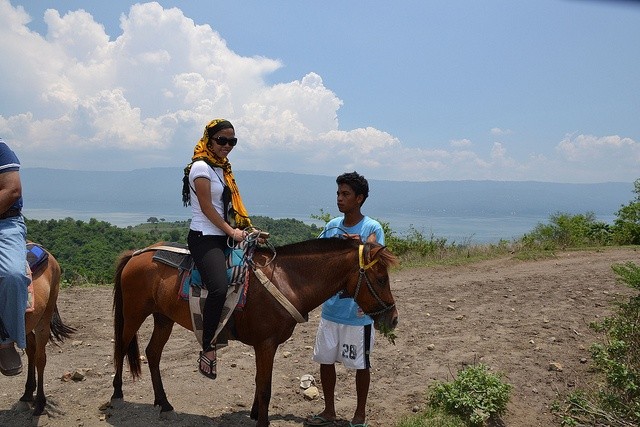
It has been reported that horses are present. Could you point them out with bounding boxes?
[19,240,80,427]
[111,237,402,427]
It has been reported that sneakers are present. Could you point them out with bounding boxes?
[0,342,23,376]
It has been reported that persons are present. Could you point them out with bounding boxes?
[183,118,265,380]
[0,137,31,376]
[303,171,386,427]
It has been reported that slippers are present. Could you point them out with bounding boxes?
[349,422,366,427]
[199,351,217,380]
[304,413,338,426]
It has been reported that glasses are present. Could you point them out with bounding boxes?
[209,136,238,146]
